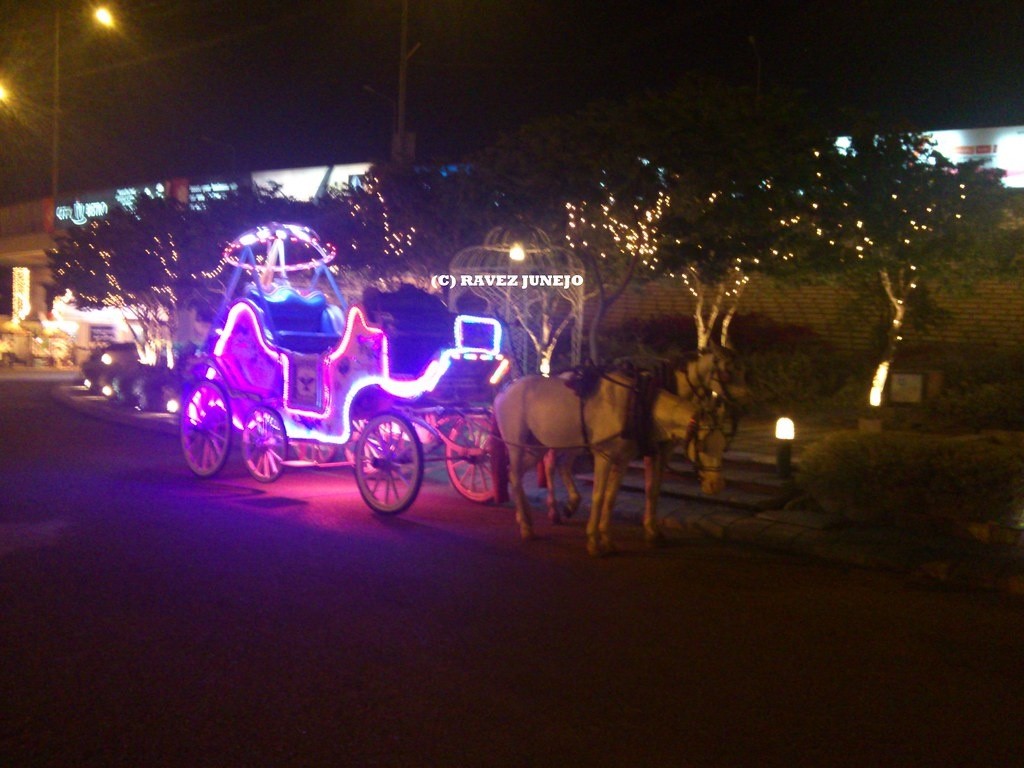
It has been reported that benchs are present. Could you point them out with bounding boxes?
[366,284,455,339]
[243,285,340,353]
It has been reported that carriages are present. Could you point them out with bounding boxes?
[173,296,756,559]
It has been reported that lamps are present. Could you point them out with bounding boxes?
[775,417,796,442]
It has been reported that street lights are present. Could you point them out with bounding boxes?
[0,0,115,254]
[508,236,550,377]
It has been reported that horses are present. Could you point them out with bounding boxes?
[489,374,731,556]
[534,337,755,517]
